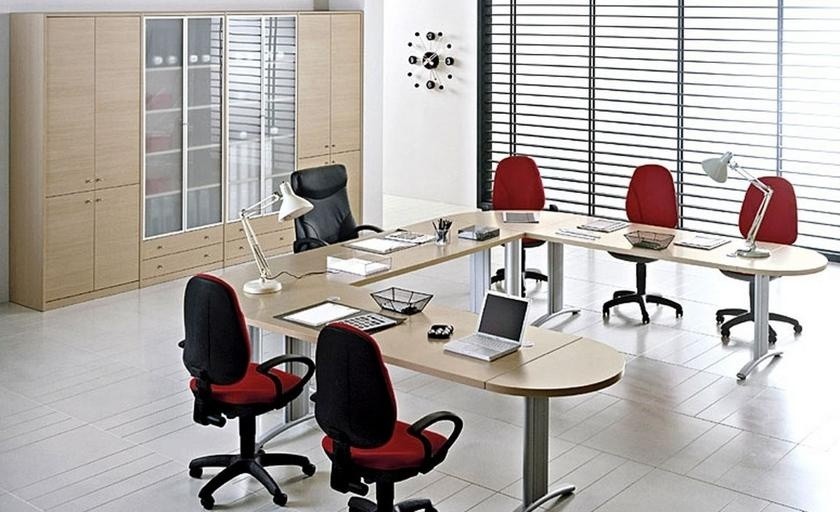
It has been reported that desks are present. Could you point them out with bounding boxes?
[210,206,829,511]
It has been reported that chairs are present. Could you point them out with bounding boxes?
[481,153,560,302]
[601,162,685,326]
[306,321,466,511]
[289,162,385,256]
[177,270,320,510]
[713,173,806,344]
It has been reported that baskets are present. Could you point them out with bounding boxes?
[370,286,433,315]
[623,229,675,251]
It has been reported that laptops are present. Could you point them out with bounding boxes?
[442,289,532,362]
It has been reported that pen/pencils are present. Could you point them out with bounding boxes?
[432,219,452,242]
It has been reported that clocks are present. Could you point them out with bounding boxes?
[400,17,459,96]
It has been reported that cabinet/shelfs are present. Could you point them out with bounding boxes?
[226,12,297,265]
[138,15,226,288]
[6,12,138,312]
[300,13,367,239]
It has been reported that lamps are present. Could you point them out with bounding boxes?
[702,149,778,260]
[234,180,317,296]
